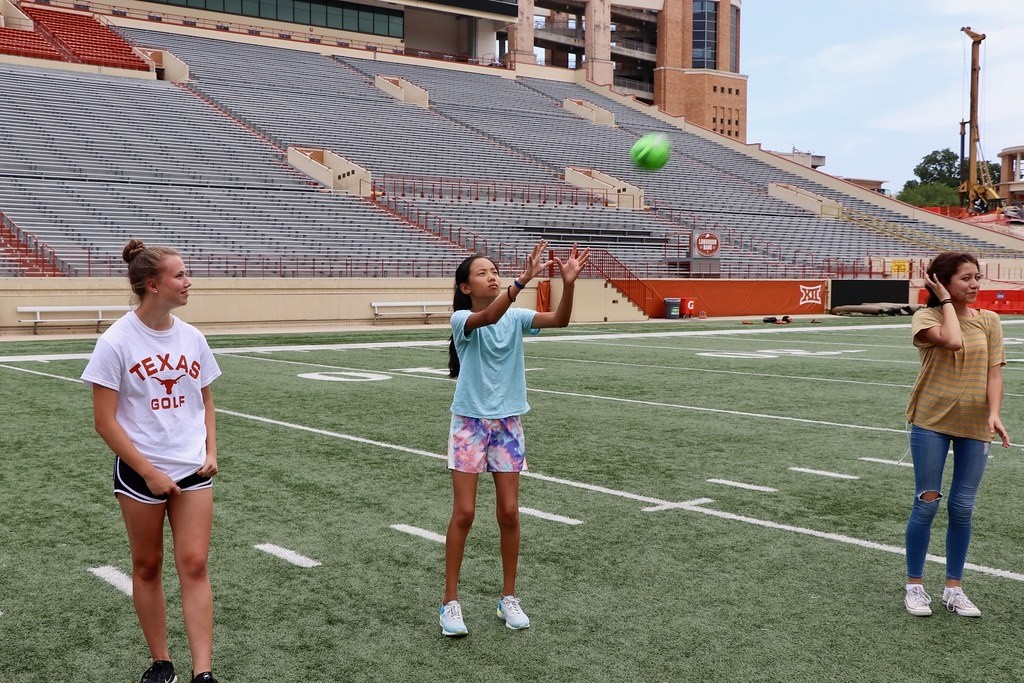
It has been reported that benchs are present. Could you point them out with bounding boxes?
[370,301,454,326]
[16,305,138,335]
[0,24,1024,277]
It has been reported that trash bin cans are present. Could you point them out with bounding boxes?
[680,298,696,318]
[664,298,681,319]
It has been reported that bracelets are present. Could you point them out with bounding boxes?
[940,299,955,305]
[508,285,515,302]
[514,280,525,289]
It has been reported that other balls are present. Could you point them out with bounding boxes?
[631,134,672,171]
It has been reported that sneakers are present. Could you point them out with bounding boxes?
[140,660,177,683]
[439,600,469,636]
[497,594,530,630]
[941,585,981,616]
[190,670,218,683]
[904,586,933,616]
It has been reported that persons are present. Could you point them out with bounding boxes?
[81,239,222,683]
[905,251,1010,617]
[439,239,591,635]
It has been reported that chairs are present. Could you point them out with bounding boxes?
[0,0,162,72]
